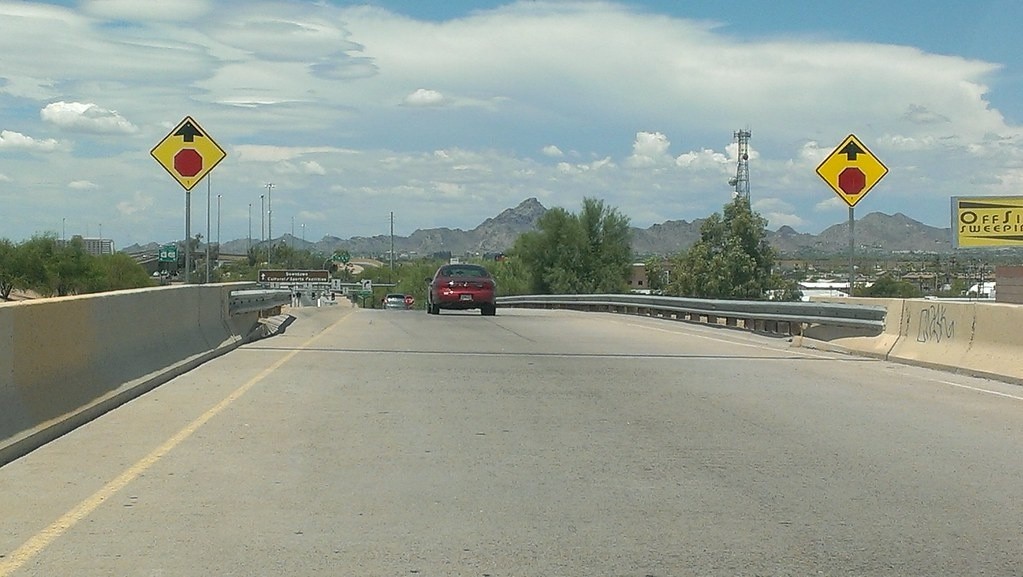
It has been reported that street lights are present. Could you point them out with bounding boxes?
[264,183,276,266]
[259,193,265,246]
[216,194,222,253]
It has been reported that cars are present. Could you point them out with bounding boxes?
[424,264,497,316]
[382,294,408,311]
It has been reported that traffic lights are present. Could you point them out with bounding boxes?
[331,293,335,301]
[312,292,316,300]
